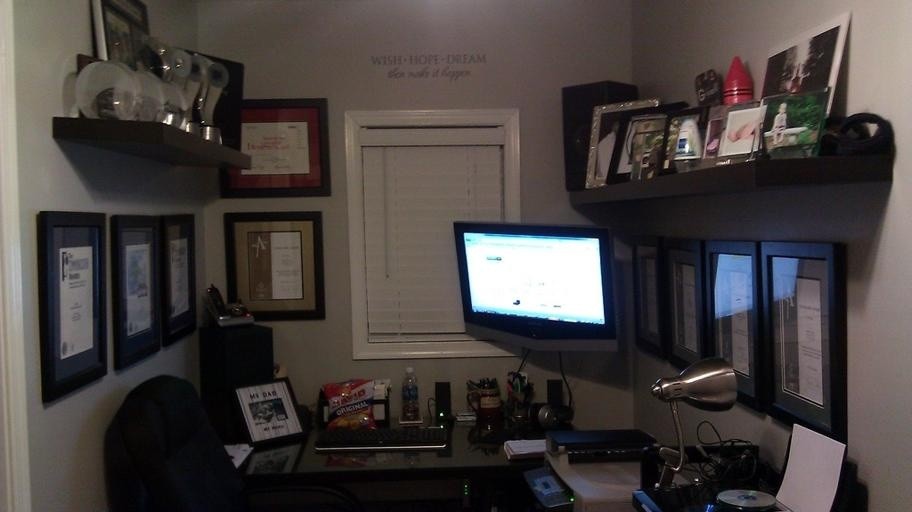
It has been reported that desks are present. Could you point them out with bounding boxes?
[240,413,580,512]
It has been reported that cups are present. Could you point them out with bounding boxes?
[466,387,501,421]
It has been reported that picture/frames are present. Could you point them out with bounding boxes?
[216,95,332,198]
[35,210,198,403]
[221,210,327,322]
[232,377,308,453]
[630,234,848,444]
[584,10,851,190]
[89,0,150,71]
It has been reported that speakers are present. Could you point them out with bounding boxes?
[547,378,564,418]
[197,324,273,442]
[435,381,457,426]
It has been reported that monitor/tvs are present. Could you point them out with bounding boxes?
[713,253,800,321]
[453,220,619,352]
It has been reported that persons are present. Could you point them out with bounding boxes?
[770,102,788,145]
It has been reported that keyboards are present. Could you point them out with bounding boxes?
[313,424,451,452]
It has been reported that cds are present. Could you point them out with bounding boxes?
[720,488,778,508]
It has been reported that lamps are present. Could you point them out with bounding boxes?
[646,356,738,490]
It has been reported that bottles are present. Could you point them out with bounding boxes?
[401,367,419,421]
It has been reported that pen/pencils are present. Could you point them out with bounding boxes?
[466,377,498,389]
[506,372,523,393]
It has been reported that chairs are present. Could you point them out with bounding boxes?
[103,375,360,512]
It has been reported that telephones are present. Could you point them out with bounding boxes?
[205,283,254,326]
